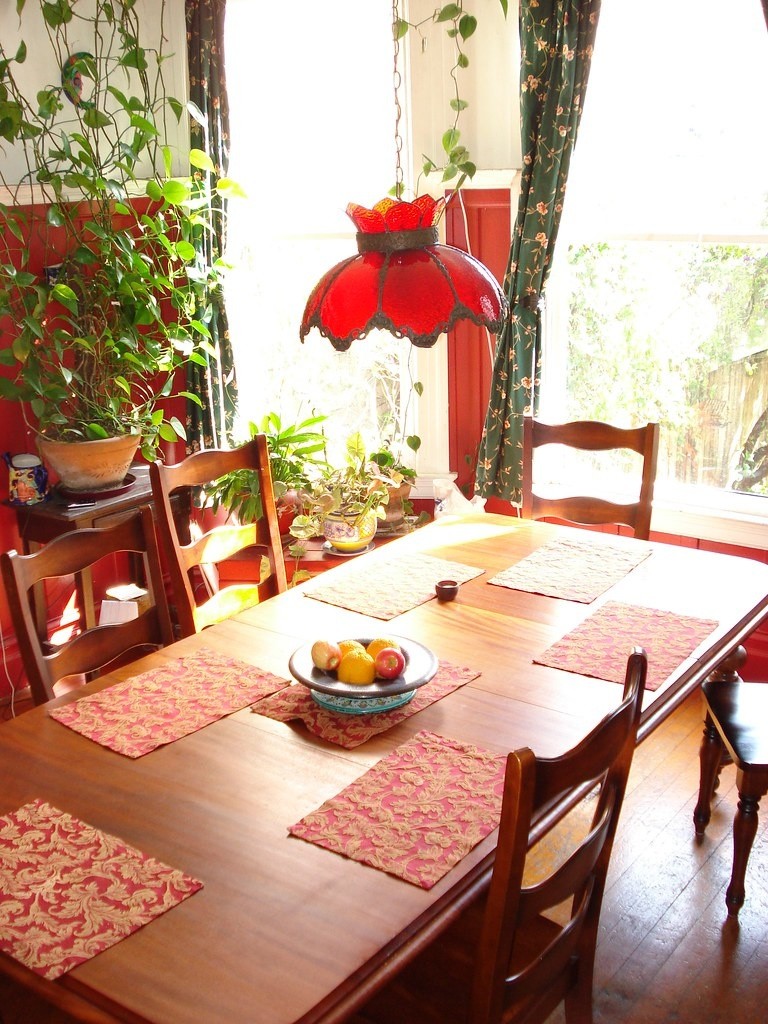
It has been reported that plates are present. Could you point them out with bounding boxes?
[376,516,420,536]
[321,540,375,557]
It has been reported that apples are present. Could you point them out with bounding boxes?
[312,639,343,671]
[375,647,406,679]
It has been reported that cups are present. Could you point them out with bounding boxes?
[3,452,50,506]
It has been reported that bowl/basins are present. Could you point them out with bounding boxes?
[435,580,459,601]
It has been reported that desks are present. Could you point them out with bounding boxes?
[0,461,175,657]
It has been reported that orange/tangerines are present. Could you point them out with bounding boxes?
[336,637,402,685]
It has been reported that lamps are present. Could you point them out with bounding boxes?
[299,0,510,352]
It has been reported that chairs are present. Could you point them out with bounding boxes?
[150,434,287,637]
[0,513,768,1024]
[522,416,660,541]
[344,645,648,1023]
[692,681,768,919]
[0,504,175,712]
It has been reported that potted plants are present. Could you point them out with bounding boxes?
[341,431,420,533]
[0,0,245,487]
[289,467,390,552]
